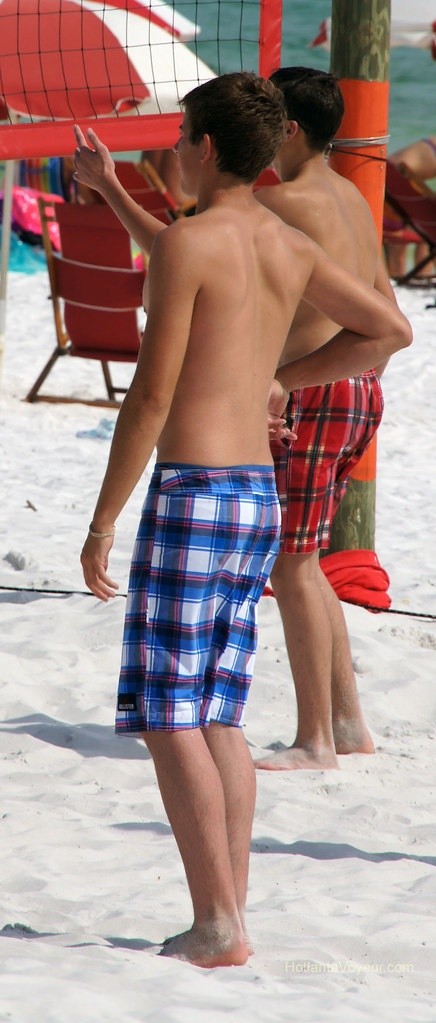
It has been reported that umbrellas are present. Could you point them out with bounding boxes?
[1,1,223,329]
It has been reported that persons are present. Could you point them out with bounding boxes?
[82,73,413,965]
[386,128,436,275]
[68,66,401,770]
[142,145,190,207]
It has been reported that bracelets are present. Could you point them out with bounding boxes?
[88,523,116,538]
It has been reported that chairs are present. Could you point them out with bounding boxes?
[27,198,149,402]
[389,152,434,309]
[87,161,188,226]
